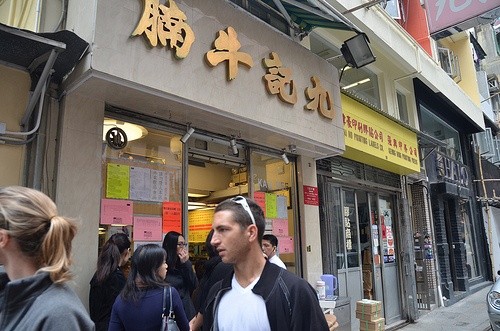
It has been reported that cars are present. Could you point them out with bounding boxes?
[486,270,500,331]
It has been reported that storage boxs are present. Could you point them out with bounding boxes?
[355,299,385,331]
[232,172,247,183]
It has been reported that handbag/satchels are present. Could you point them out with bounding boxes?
[160,285,180,331]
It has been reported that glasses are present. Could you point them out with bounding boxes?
[177,242,187,248]
[230,195,256,224]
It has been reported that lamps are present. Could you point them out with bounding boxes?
[188,147,246,167]
[229,134,238,155]
[340,33,376,70]
[280,147,290,165]
[180,121,195,144]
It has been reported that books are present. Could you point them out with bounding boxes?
[359,223,369,243]
[346,223,358,253]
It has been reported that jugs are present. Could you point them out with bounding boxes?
[320,275,338,297]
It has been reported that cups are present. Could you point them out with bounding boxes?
[316,281,326,300]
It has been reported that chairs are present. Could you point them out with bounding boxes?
[319,300,339,331]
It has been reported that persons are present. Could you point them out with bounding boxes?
[191,194,328,331]
[187,229,236,331]
[106,242,191,331]
[262,234,287,270]
[161,231,199,331]
[0,184,98,331]
[89,233,131,331]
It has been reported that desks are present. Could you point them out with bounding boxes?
[319,296,351,331]
